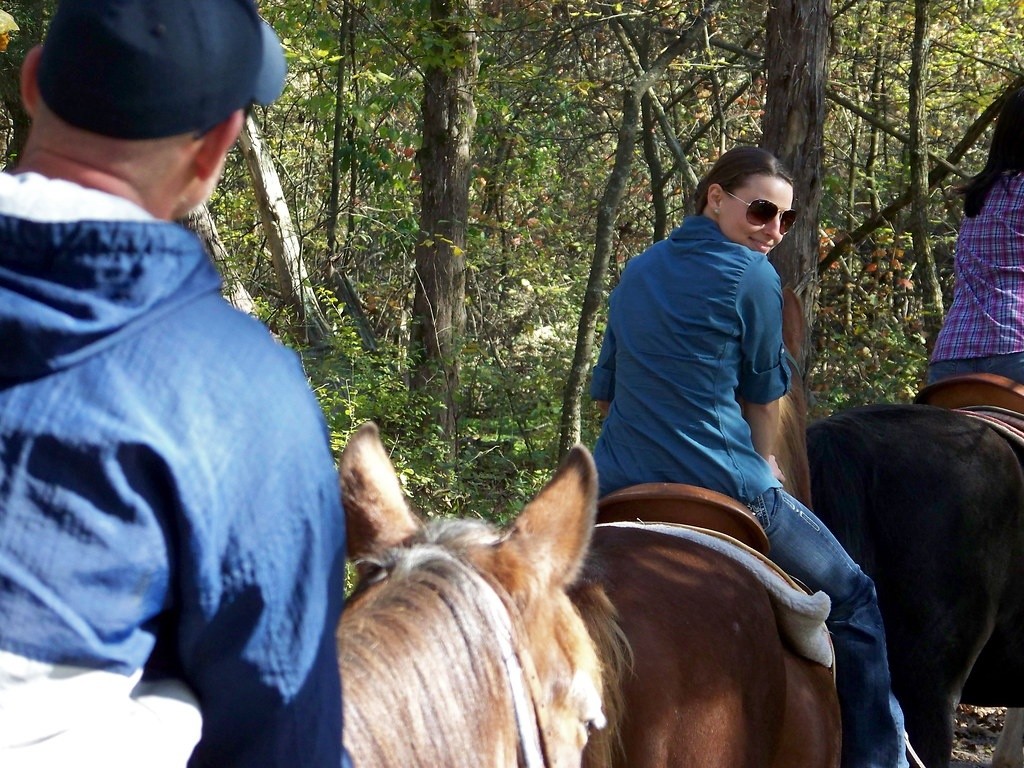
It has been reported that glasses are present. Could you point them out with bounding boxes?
[726,191,798,236]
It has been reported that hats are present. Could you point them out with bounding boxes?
[36,0,285,141]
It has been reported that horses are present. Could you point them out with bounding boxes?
[804,401,1024,768]
[332,420,635,767]
[565,284,841,768]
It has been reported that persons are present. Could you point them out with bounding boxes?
[0,0,354,768]
[923,83,1024,388]
[586,143,913,768]
